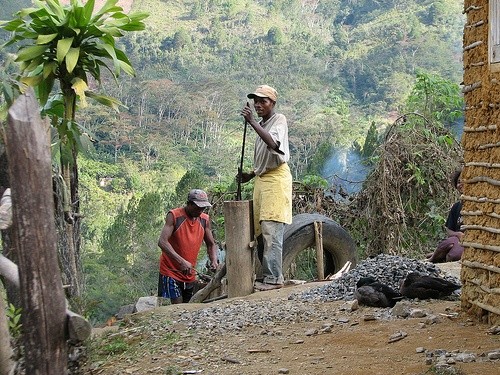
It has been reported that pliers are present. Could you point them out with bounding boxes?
[188,266,204,278]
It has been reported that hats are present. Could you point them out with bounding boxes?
[247,85,279,103]
[188,189,212,207]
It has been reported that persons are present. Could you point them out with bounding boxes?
[157,189,220,305]
[425,170,465,262]
[234,85,293,285]
[0,152,13,231]
